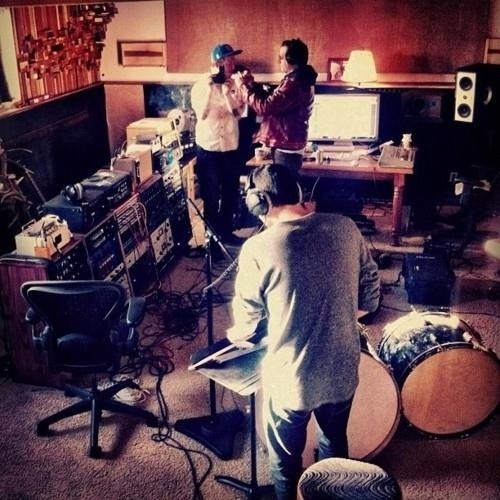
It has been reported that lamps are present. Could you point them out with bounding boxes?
[341,49,378,86]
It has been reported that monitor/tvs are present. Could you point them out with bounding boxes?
[307,93,381,153]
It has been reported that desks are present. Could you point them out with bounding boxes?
[245,155,414,247]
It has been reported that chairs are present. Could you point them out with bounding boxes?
[20,279,158,458]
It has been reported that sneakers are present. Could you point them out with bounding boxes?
[222,233,242,247]
[250,485,277,500]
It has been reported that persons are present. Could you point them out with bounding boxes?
[223,158,382,500]
[228,36,319,184]
[189,43,258,250]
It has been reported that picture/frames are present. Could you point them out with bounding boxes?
[326,57,349,83]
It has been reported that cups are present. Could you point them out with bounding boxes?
[255,149,268,162]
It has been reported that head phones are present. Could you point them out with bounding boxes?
[61,182,85,206]
[245,165,310,216]
[285,39,298,65]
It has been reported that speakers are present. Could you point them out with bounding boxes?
[454,62,497,124]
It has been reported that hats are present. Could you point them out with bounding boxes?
[211,43,242,63]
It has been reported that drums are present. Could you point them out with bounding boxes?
[377,309,500,440]
[255,315,402,470]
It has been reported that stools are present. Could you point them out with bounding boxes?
[296,456,403,499]
